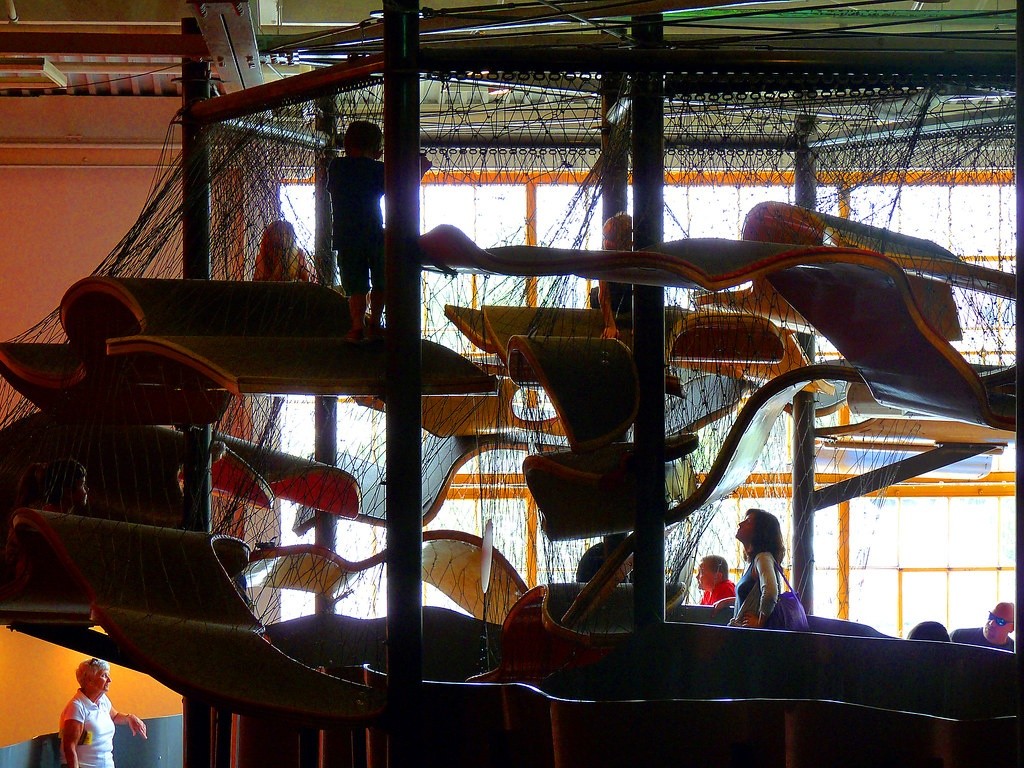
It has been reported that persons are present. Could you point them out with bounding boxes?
[577,533,629,582]
[6,458,88,582]
[696,557,736,605]
[251,221,308,282]
[909,621,950,641]
[953,602,1014,651]
[712,510,783,629]
[590,214,632,338]
[59,659,147,768]
[326,121,432,341]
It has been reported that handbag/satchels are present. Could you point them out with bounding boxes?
[754,557,809,631]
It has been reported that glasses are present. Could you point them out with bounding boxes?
[84,658,98,676]
[988,612,1014,626]
